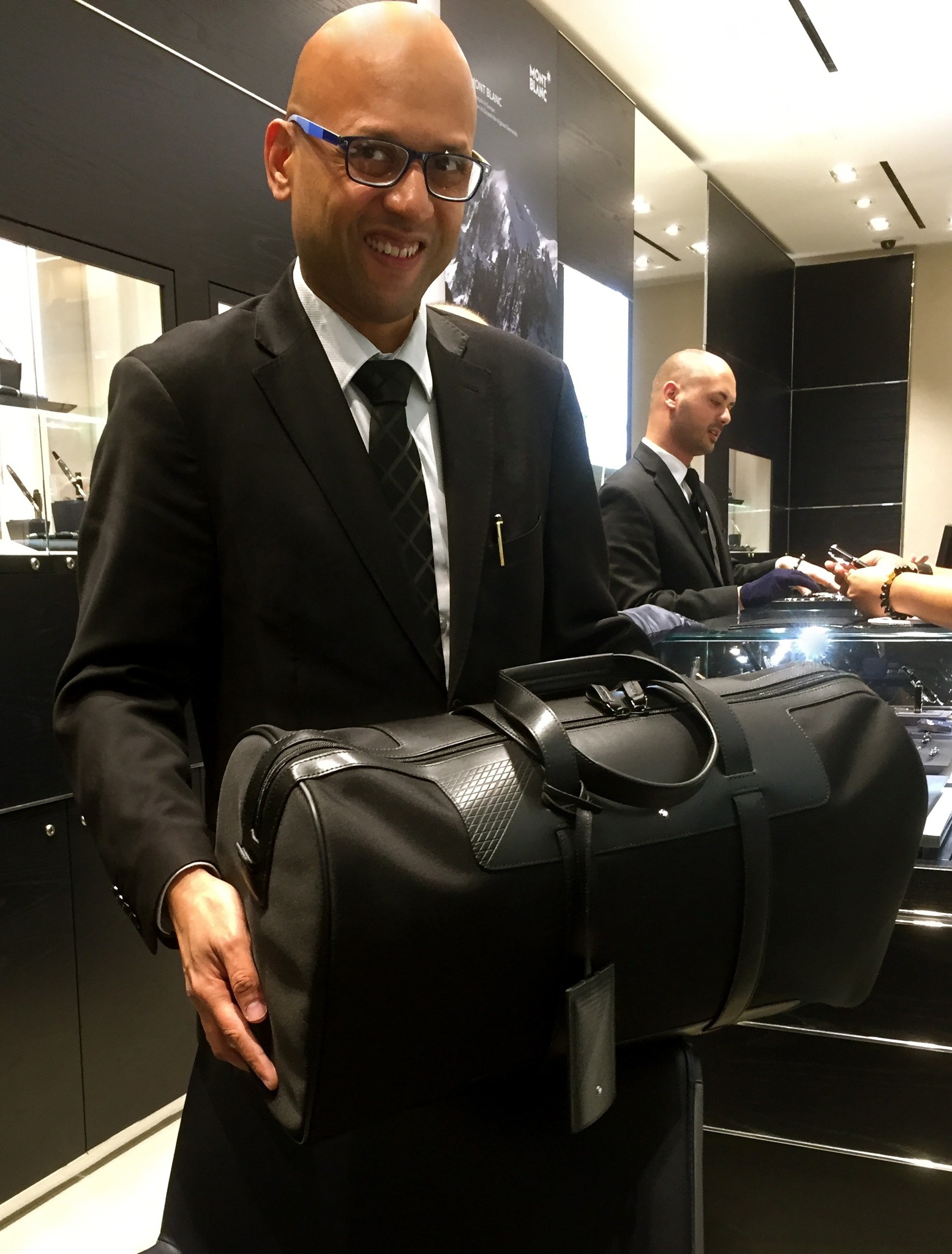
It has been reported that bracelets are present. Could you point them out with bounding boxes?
[914,563,933,576]
[879,565,917,620]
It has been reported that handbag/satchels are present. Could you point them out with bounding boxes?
[217,656,929,1150]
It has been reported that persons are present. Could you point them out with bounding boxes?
[42,1,660,1254]
[598,349,839,683]
[824,549,952,634]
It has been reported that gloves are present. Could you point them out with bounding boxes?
[740,568,819,608]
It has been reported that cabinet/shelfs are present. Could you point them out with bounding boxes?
[0,554,206,1204]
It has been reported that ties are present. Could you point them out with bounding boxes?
[684,468,716,568]
[353,359,446,698]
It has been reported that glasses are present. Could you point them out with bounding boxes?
[288,115,491,202]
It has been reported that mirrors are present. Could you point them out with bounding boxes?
[630,107,710,485]
